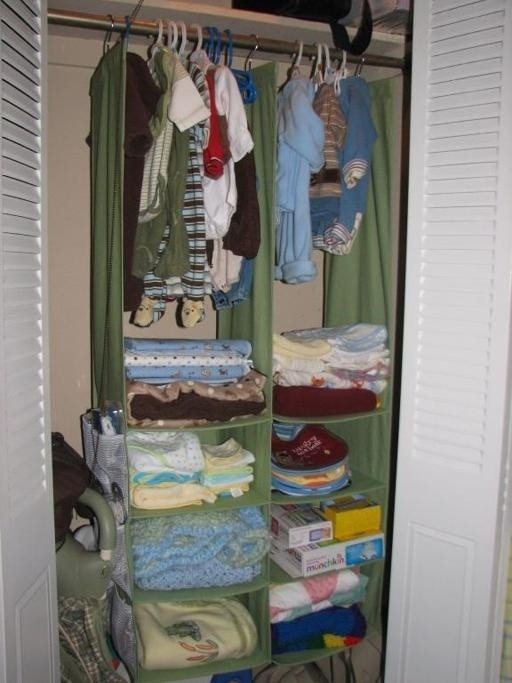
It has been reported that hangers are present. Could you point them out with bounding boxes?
[102,11,367,99]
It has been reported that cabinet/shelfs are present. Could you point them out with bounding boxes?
[87,381,392,683]
